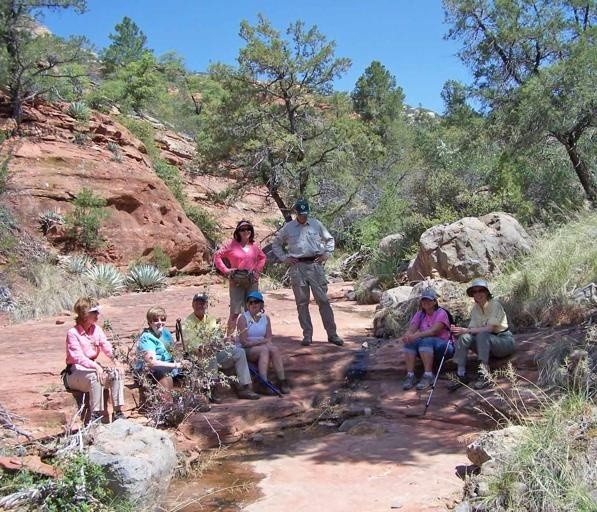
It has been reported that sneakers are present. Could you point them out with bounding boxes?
[417,375,434,389]
[475,378,488,389]
[212,395,223,403]
[114,413,127,420]
[257,379,290,395]
[403,374,416,390]
[92,415,100,425]
[328,335,343,345]
[446,375,470,390]
[301,337,312,346]
[237,390,259,400]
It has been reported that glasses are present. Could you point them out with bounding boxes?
[249,300,258,304]
[152,317,166,322]
[472,288,487,294]
[239,227,251,232]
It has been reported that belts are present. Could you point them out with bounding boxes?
[297,256,317,262]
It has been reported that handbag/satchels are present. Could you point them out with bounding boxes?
[228,269,253,284]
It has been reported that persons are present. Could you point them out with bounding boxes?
[444,278,516,391]
[401,289,458,390]
[180,291,260,403]
[272,200,346,347]
[212,219,268,346]
[60,295,133,425]
[133,305,210,413]
[236,290,291,397]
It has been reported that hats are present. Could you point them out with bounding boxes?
[466,277,487,297]
[238,222,252,227]
[246,291,264,303]
[89,305,104,312]
[295,202,309,215]
[420,288,436,300]
[193,293,207,301]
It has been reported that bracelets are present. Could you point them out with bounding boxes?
[467,327,472,335]
